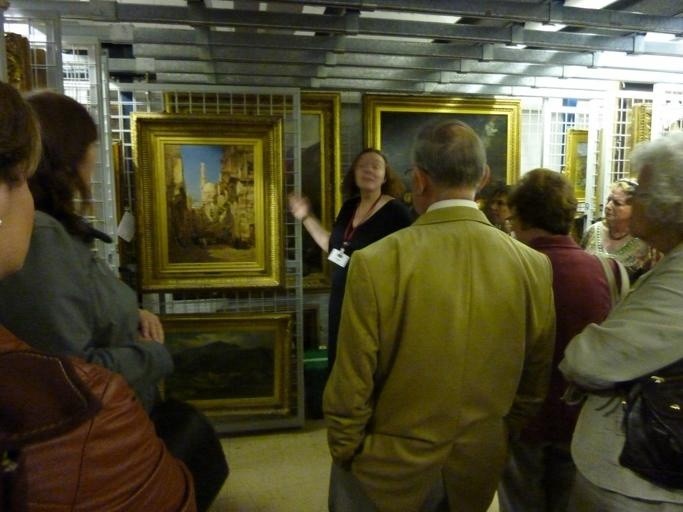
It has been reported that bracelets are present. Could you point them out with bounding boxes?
[301,212,313,223]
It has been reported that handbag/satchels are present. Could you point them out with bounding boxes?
[615,370,683,493]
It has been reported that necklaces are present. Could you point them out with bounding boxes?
[608,223,630,240]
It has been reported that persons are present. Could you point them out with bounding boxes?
[1,87,176,417]
[491,186,518,240]
[551,131,683,511]
[286,143,414,366]
[321,114,556,512]
[503,164,632,512]
[581,177,665,291]
[1,80,198,511]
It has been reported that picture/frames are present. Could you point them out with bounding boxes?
[625,102,682,193]
[5,29,34,95]
[563,125,593,205]
[127,109,287,296]
[213,301,323,353]
[161,84,346,295]
[359,89,524,236]
[67,139,124,270]
[147,308,294,424]
